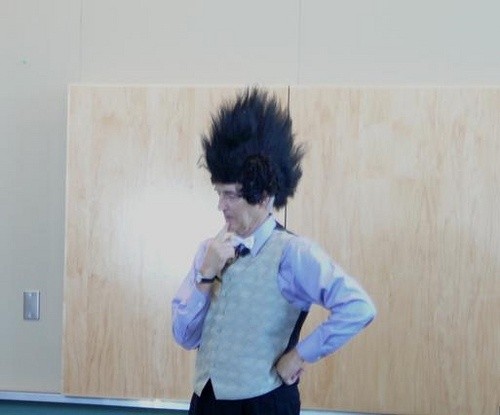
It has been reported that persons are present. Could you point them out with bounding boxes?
[168,167,377,415]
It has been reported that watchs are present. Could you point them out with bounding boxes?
[194,272,217,284]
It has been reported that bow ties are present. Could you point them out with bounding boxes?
[229,234,256,250]
